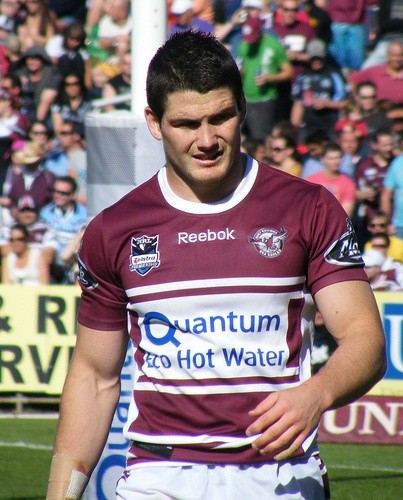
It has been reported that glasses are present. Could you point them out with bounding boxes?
[33,131,47,136]
[371,223,386,228]
[52,189,70,195]
[60,131,74,136]
[65,81,79,87]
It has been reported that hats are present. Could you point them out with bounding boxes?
[17,194,35,210]
[169,0,194,14]
[241,17,263,43]
[307,39,327,60]
[12,142,40,164]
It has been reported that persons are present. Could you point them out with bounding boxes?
[165,0,403,293]
[0,0,133,286]
[44,30,389,500]
